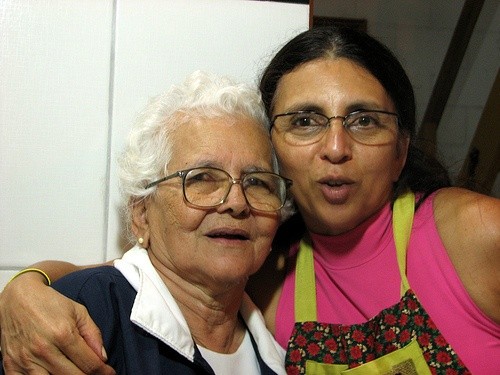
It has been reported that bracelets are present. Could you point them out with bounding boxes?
[3,269,51,290]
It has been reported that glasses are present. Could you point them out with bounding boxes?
[144,166,294,213]
[269,110,403,146]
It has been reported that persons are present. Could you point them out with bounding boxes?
[1,24,499,375]
[0,74,286,375]
